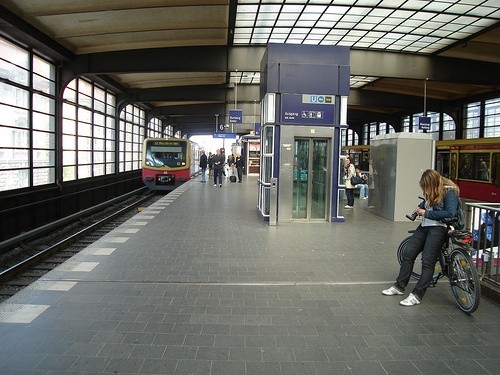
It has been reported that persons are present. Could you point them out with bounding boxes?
[355,166,369,199]
[208,148,245,188]
[382,170,465,306]
[199,150,207,182]
[343,157,356,209]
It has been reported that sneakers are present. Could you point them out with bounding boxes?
[399,292,420,306]
[381,287,406,296]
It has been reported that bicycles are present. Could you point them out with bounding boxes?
[397,197,481,314]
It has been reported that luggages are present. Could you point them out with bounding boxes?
[230,166,236,183]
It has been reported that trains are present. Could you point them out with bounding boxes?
[342,138,500,169]
[142,138,206,190]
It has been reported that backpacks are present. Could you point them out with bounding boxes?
[359,175,366,184]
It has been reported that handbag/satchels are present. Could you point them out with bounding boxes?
[351,176,359,185]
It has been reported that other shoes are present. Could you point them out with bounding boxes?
[344,205,353,208]
[214,184,217,186]
[219,184,222,187]
